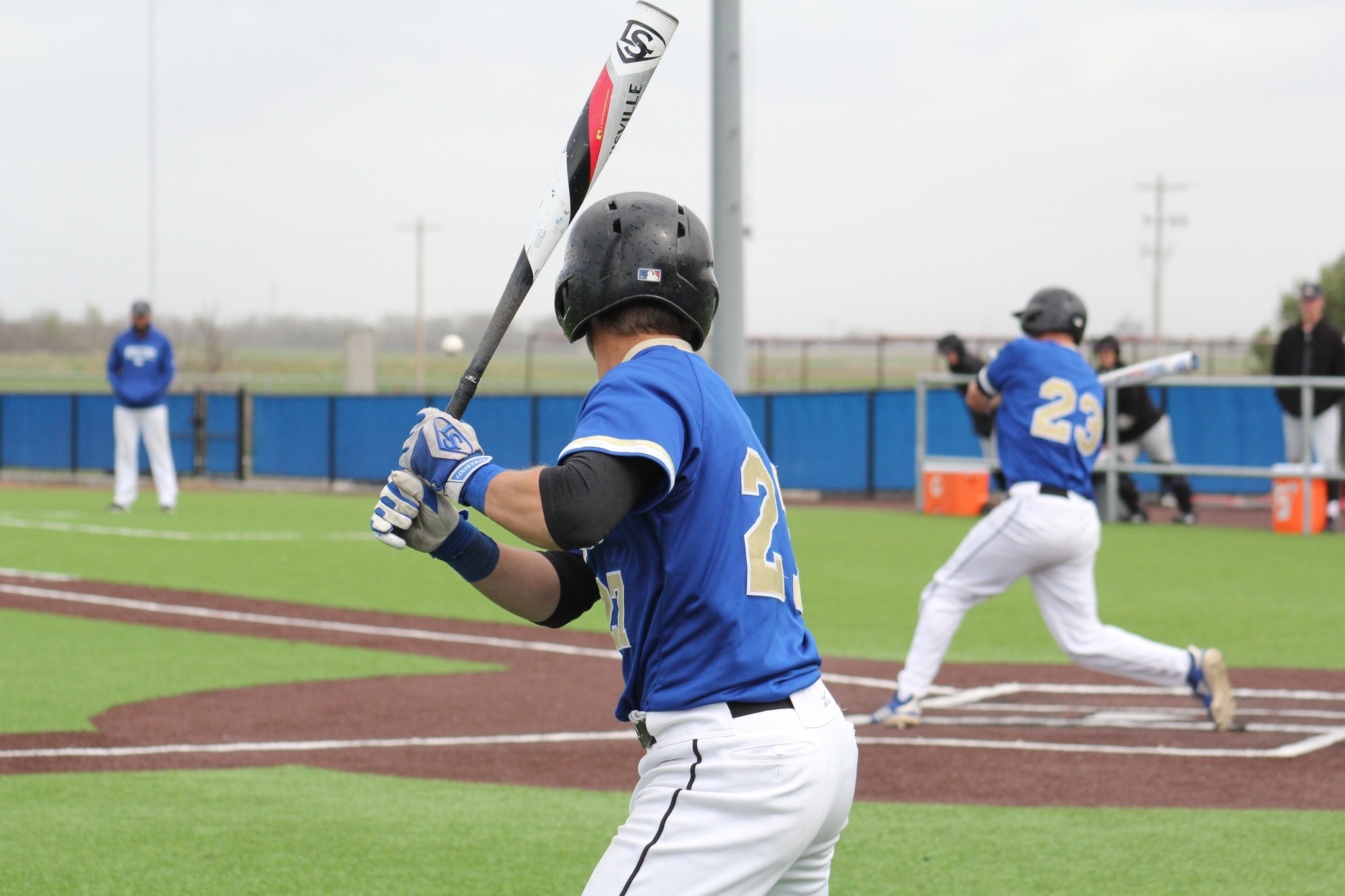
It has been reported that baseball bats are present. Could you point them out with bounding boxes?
[445,0,679,421]
[1097,351,1199,390]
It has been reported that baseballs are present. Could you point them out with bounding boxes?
[441,333,463,356]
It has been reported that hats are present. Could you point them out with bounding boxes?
[131,303,152,316]
[1301,284,1322,300]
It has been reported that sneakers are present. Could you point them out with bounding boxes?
[1186,645,1236,731]
[869,691,922,729]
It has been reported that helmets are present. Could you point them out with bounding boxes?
[1013,288,1086,344]
[1094,336,1120,355]
[554,192,719,350]
[937,335,965,362]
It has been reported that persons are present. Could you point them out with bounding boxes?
[869,290,1237,731]
[106,303,178,516]
[372,192,858,896]
[1272,284,1345,531]
[1091,337,1199,527]
[937,334,1008,492]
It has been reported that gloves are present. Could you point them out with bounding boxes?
[399,407,493,507]
[371,472,476,562]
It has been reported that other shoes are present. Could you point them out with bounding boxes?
[1179,505,1195,525]
[1130,508,1147,523]
[1326,501,1341,522]
